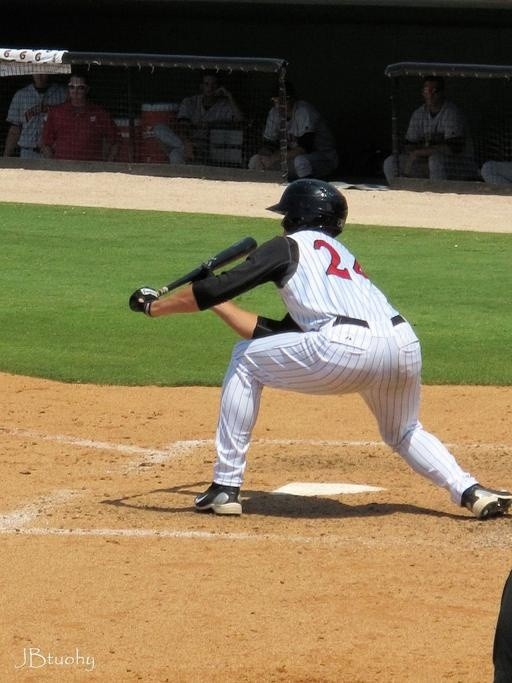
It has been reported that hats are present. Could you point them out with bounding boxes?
[271,81,295,98]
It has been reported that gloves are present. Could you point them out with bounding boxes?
[127,286,160,319]
[187,263,217,281]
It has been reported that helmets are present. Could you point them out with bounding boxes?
[265,179,349,237]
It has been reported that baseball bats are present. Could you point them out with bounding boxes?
[157,235,257,295]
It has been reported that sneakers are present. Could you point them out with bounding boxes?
[460,482,512,520]
[192,480,245,517]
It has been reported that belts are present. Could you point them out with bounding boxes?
[331,312,407,330]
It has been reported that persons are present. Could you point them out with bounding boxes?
[129,179,512,522]
[492,569,511,683]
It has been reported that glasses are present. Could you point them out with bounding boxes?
[67,83,88,90]
